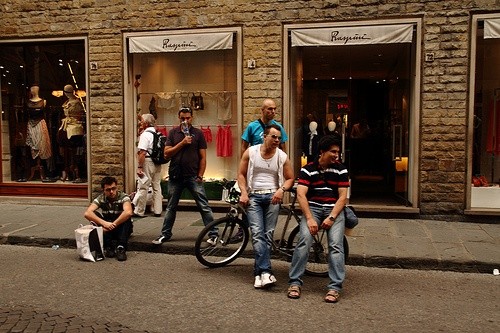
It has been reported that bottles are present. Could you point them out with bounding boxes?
[181,121,190,137]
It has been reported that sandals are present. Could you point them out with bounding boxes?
[287,284,301,298]
[324,290,342,303]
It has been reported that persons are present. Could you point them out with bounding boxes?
[150,107,219,247]
[327,121,336,131]
[287,135,350,303]
[24,86,52,182]
[84,177,134,262]
[350,115,372,138]
[235,125,295,290]
[134,113,163,218]
[227,99,288,243]
[57,85,87,182]
[302,122,322,163]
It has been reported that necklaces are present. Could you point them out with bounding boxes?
[259,144,276,167]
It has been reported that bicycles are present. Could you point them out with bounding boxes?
[195,178,350,277]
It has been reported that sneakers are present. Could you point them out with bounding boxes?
[254,275,262,288]
[117,247,126,260]
[151,234,169,244]
[261,272,277,288]
[106,247,116,258]
[206,236,218,246]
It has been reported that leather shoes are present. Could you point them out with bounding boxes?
[131,211,144,218]
[153,213,161,217]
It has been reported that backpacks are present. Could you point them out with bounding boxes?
[144,130,170,165]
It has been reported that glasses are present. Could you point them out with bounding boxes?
[266,134,281,141]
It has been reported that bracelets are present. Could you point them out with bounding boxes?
[328,215,335,221]
[280,186,286,192]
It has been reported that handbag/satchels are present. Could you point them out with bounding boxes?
[343,205,358,229]
[74,221,105,263]
